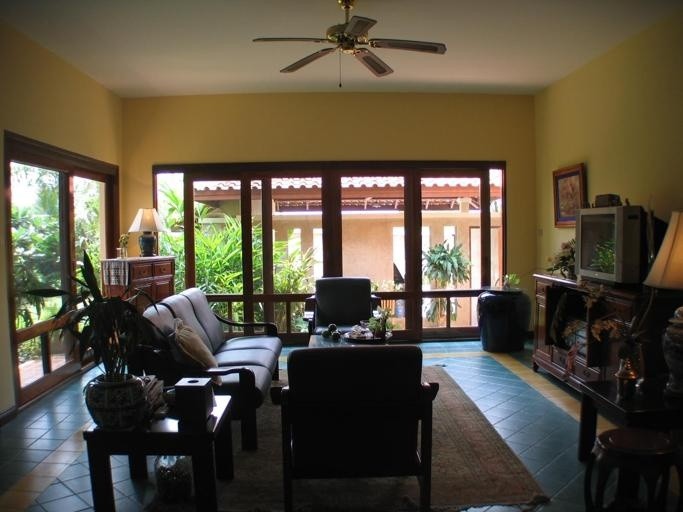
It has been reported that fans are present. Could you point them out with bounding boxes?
[252,0,447,88]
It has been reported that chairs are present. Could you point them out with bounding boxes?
[302,277,384,336]
[269,345,440,512]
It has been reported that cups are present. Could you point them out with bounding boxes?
[359,320,370,328]
[115,248,126,260]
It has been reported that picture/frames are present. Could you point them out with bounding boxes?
[552,163,588,229]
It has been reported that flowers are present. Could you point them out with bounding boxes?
[372,305,391,320]
[543,237,576,279]
[549,280,652,371]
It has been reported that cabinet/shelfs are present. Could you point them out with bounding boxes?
[100,256,178,316]
[532,271,682,395]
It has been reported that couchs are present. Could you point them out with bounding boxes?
[127,286,283,452]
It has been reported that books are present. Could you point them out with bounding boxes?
[142,373,164,414]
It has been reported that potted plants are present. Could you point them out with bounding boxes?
[20,249,163,429]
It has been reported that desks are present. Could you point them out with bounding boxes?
[82,394,236,512]
[576,378,683,501]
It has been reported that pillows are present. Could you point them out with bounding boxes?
[172,317,224,386]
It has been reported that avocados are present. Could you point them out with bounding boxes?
[321,324,341,340]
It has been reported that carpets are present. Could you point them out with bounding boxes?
[141,363,552,512]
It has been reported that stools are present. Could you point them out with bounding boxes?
[582,428,683,512]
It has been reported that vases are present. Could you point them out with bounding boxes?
[566,263,577,280]
[660,306,682,397]
[380,319,387,345]
[619,351,640,384]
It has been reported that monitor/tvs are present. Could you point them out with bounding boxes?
[575,205,668,292]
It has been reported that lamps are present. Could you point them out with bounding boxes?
[642,209,682,291]
[127,206,172,257]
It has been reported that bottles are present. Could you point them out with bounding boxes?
[614,357,638,403]
